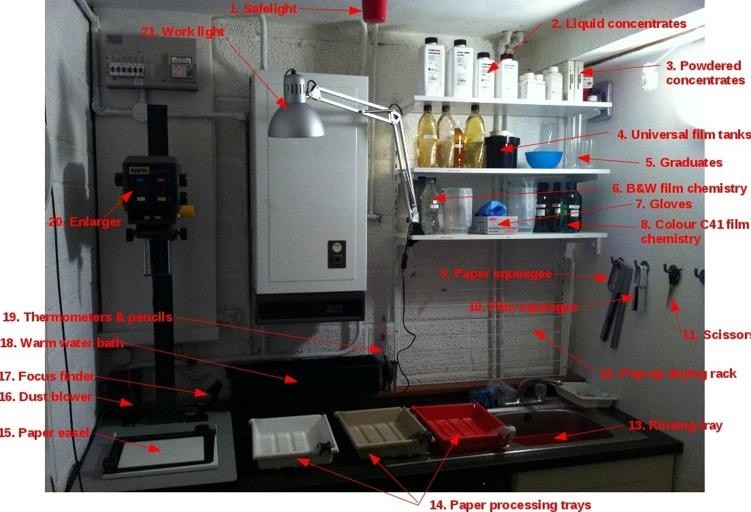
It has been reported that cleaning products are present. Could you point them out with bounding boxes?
[417,37,564,100]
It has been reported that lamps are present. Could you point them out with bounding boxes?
[268,67,424,236]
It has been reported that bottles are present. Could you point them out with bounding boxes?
[534,178,583,234]
[416,35,563,101]
[416,103,486,169]
[414,174,443,235]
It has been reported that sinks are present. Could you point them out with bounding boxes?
[495,409,615,447]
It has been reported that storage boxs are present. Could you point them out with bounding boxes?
[468,215,518,235]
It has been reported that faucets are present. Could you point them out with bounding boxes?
[515,376,563,403]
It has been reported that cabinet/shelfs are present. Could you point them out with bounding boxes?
[398,95,613,264]
[494,456,676,491]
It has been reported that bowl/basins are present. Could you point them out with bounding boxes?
[524,150,566,168]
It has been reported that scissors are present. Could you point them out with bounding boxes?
[666,265,681,308]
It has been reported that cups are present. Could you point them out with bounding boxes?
[440,187,473,235]
[504,187,537,233]
[486,135,521,168]
